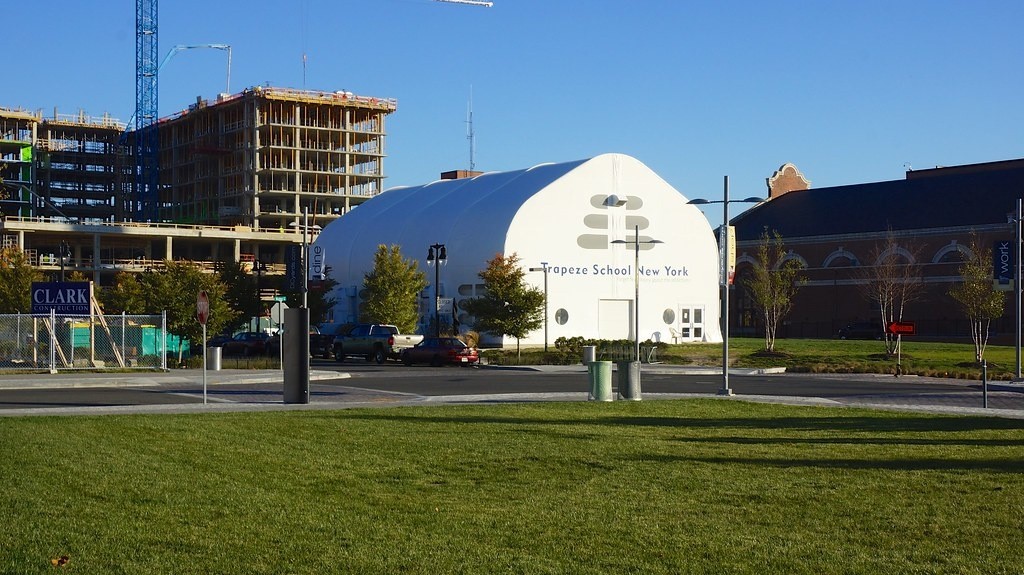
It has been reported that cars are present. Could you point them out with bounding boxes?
[222,332,270,358]
[400,337,478,368]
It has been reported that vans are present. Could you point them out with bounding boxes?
[839,320,886,341]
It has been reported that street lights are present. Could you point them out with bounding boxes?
[609,225,665,362]
[427,242,449,338]
[528,262,550,351]
[288,207,323,309]
[1006,198,1024,381]
[685,175,768,399]
[57,238,72,281]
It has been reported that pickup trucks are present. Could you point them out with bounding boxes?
[333,323,425,364]
[263,324,338,359]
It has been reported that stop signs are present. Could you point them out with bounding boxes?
[196,289,211,325]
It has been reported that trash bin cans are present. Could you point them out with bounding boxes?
[587,361,614,402]
[207,346,223,370]
[582,345,597,365]
[616,359,643,402]
